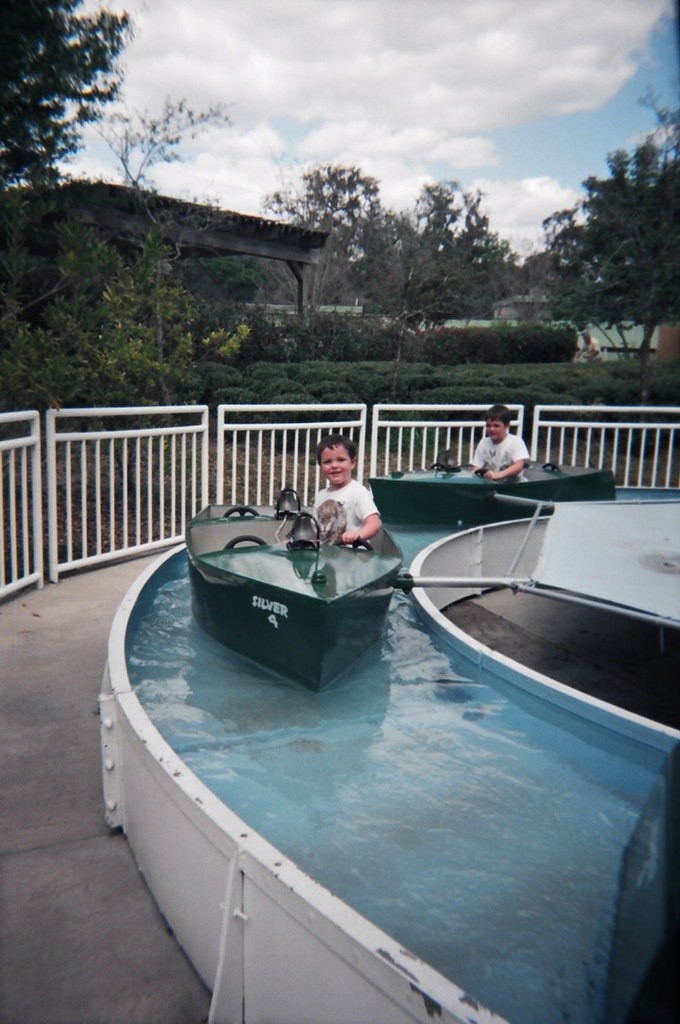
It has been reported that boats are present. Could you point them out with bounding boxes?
[366,460,621,524]
[183,503,406,691]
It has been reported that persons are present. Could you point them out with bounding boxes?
[310,434,383,549]
[468,403,532,481]
[575,331,603,362]
